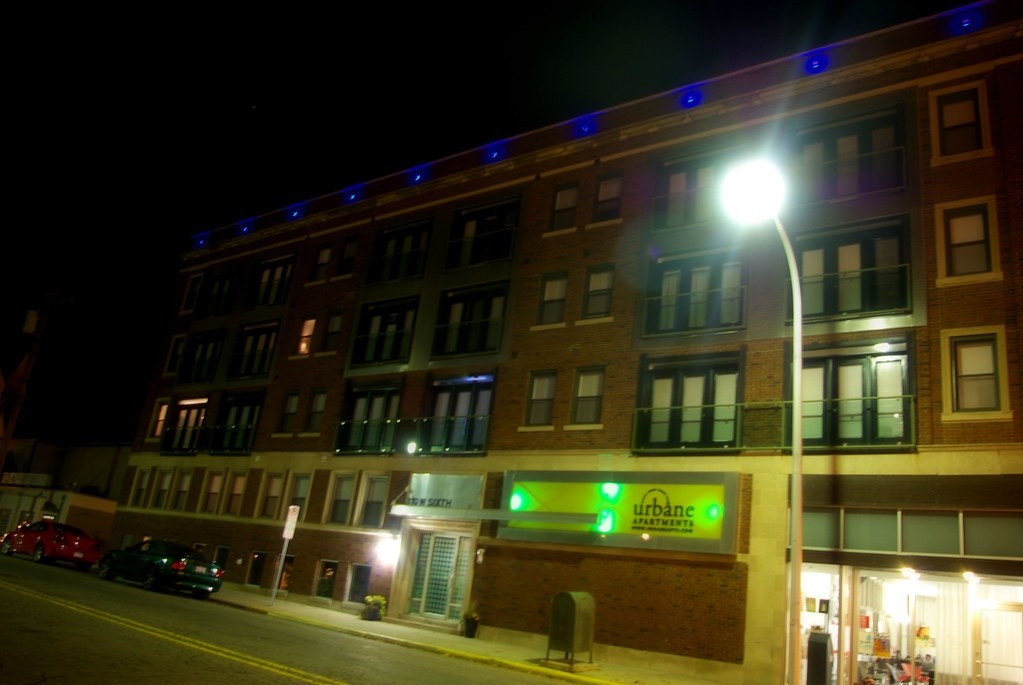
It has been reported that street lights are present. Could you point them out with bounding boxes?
[714,152,805,685]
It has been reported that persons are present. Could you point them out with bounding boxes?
[925,654,933,663]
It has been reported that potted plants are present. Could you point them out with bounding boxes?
[459,598,481,638]
[361,594,388,621]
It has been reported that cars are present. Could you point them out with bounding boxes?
[0,519,106,570]
[97,537,226,601]
